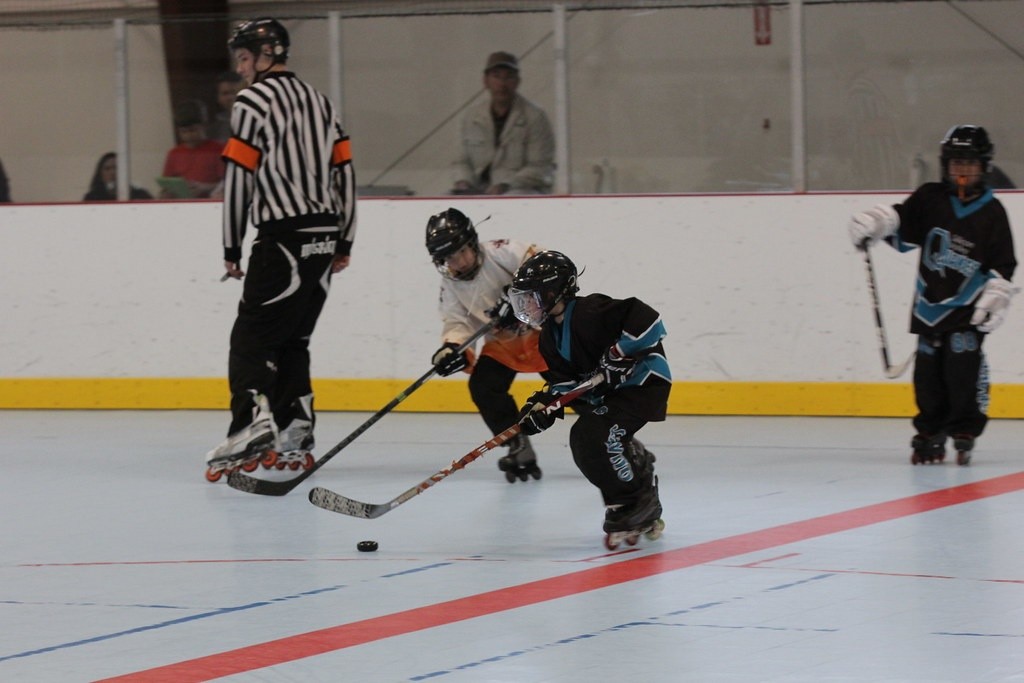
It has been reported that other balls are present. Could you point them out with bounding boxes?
[356,540,379,552]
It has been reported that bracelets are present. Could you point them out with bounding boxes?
[500,182,510,192]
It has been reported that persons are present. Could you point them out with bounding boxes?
[448,51,557,195]
[426,208,656,483]
[510,249,673,550]
[1,70,246,201]
[851,126,1017,467]
[206,16,359,482]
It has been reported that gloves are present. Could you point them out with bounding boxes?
[849,203,900,250]
[432,342,467,377]
[595,347,636,394]
[484,284,531,336]
[512,391,564,436]
[970,279,1014,332]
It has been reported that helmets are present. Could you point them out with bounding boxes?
[426,208,476,260]
[512,250,579,299]
[939,124,994,196]
[227,17,292,47]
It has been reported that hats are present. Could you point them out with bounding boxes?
[485,52,518,71]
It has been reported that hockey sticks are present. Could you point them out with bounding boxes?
[226,320,497,497]
[306,371,608,520]
[864,246,919,380]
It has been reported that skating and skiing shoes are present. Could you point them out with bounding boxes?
[912,433,947,464]
[497,436,541,483]
[955,431,974,465]
[206,413,277,482]
[603,475,665,551]
[262,396,316,471]
[626,438,655,477]
